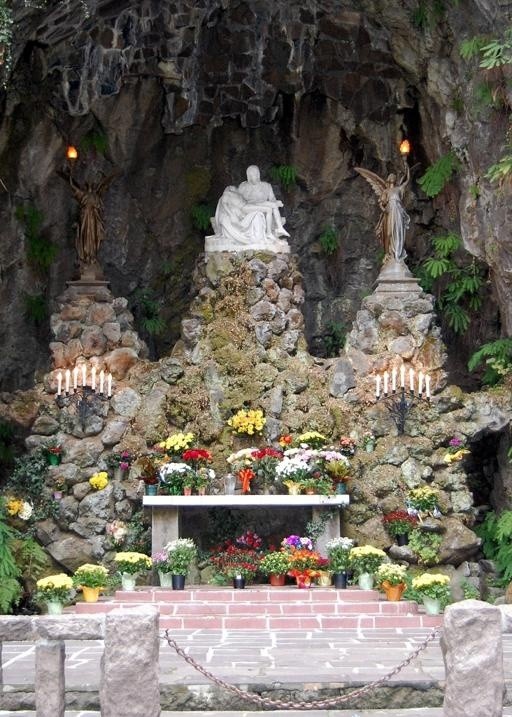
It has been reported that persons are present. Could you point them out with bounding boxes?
[355,158,420,262]
[204,164,292,253]
[58,167,123,280]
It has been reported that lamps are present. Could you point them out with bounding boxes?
[68,147,79,175]
[398,139,409,175]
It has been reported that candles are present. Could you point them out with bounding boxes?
[56,362,113,401]
[374,363,431,401]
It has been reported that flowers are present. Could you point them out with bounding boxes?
[111,408,355,494]
[37,564,110,618]
[112,532,388,591]
[89,472,108,489]
[375,563,451,617]
[382,438,471,547]
[4,439,68,529]
